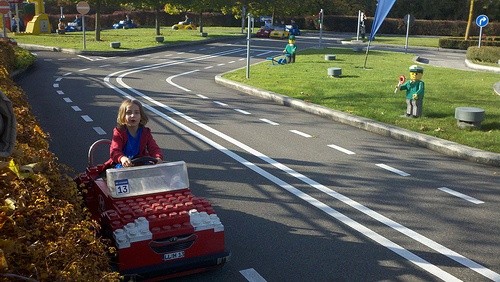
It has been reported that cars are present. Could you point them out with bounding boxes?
[256,23,301,39]
[72,139,233,282]
[112,19,138,29]
[65,22,82,32]
[171,21,197,31]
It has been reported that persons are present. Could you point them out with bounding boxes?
[124,14,131,24]
[95,99,164,190]
[394,65,424,117]
[182,14,194,24]
[12,14,19,32]
[282,35,297,63]
[74,15,82,25]
[264,19,297,30]
